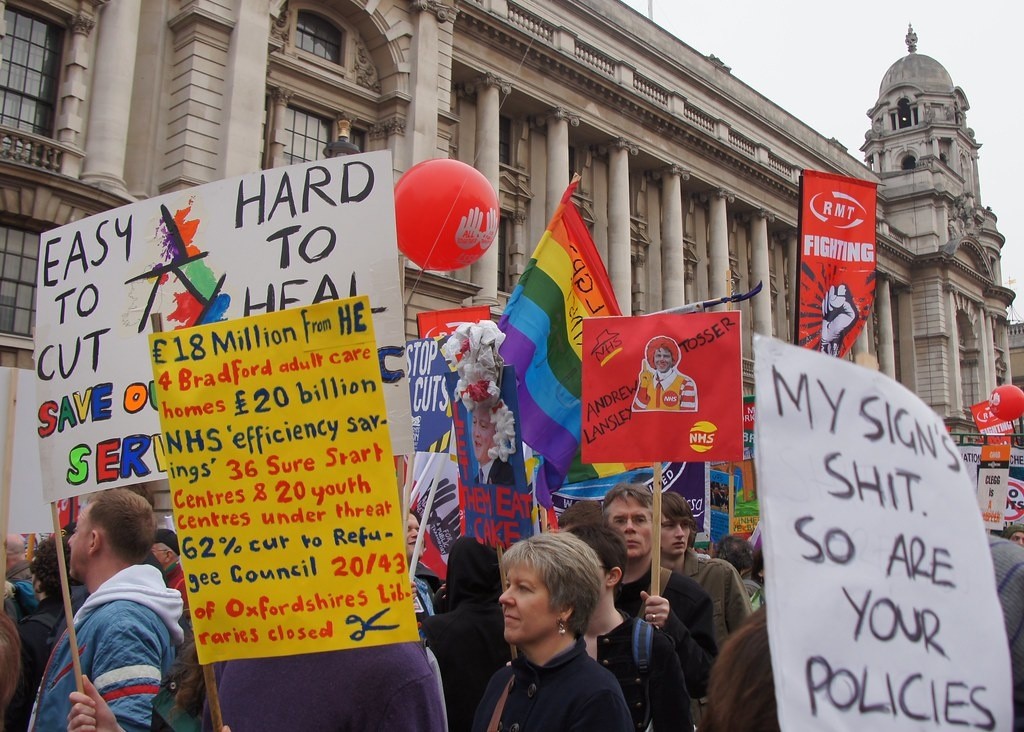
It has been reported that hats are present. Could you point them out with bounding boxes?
[1001,522,1024,540]
[154,529,180,556]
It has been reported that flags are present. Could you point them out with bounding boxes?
[489,181,625,507]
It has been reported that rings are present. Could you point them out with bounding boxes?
[653,614,656,621]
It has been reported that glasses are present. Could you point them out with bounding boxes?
[151,548,169,556]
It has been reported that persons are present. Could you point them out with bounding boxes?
[472,406,514,485]
[473,529,635,731]
[25,489,186,732]
[1,483,1024,732]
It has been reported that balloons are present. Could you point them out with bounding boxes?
[988,384,1024,421]
[395,156,499,270]
